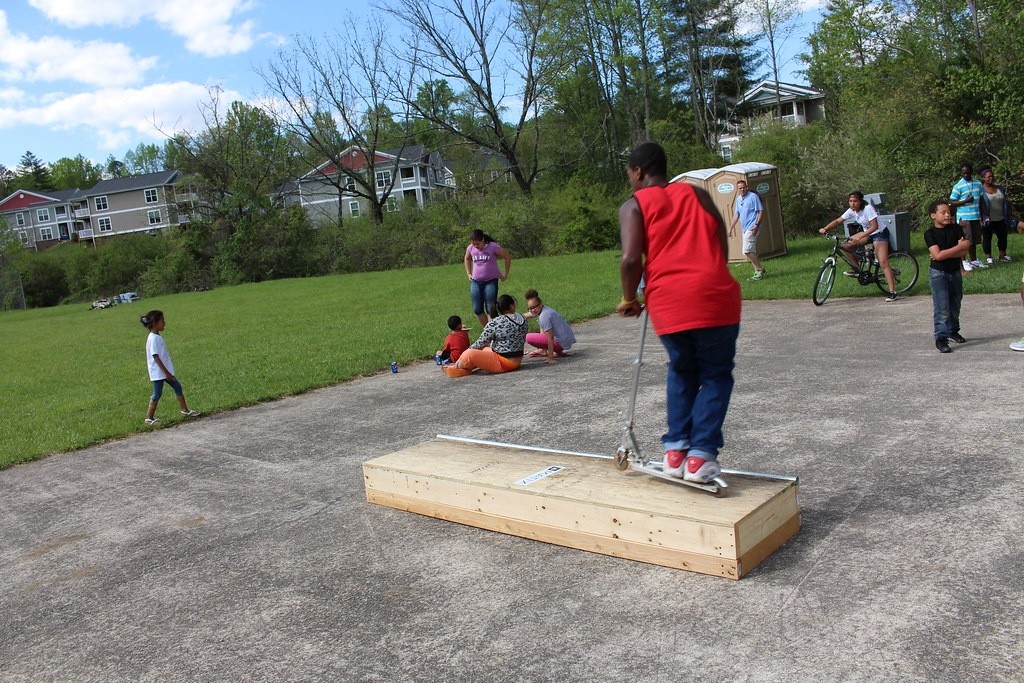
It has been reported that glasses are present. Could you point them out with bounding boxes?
[527,302,541,312]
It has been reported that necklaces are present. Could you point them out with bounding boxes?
[966,179,974,195]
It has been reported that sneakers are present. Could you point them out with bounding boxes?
[949,332,965,344]
[683,455,720,483]
[886,291,898,302]
[962,259,973,272]
[971,258,986,269]
[1009,338,1024,352]
[843,270,860,277]
[935,338,951,353]
[663,448,687,478]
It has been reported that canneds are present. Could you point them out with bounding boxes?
[982,218,990,228]
[391,361,399,373]
[1010,219,1016,228]
[435,355,449,366]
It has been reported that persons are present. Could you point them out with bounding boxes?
[442,294,529,376]
[949,165,1012,271]
[524,290,576,364]
[728,180,767,281]
[437,316,471,363]
[1009,222,1024,351]
[464,229,510,328]
[141,311,200,426]
[924,199,971,353]
[617,144,742,484]
[819,191,899,301]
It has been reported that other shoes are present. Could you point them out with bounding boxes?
[752,268,766,281]
[986,258,994,264]
[999,254,1009,263]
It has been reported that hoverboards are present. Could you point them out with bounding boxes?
[614,303,729,498]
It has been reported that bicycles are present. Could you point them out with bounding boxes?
[812,230,920,307]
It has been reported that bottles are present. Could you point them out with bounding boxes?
[865,248,870,264]
[874,249,879,263]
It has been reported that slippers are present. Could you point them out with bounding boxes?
[528,351,547,356]
[553,351,568,357]
[447,367,473,378]
[442,364,452,373]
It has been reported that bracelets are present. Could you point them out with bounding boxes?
[620,295,639,305]
[755,224,759,226]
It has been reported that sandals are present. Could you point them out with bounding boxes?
[181,409,200,416]
[144,418,163,426]
